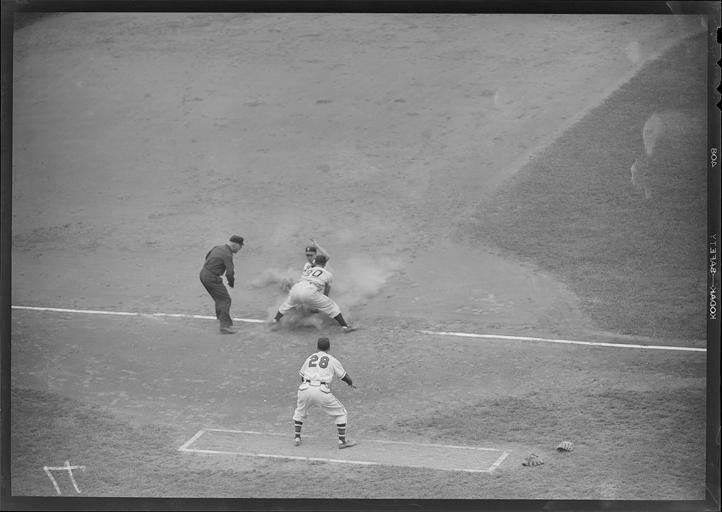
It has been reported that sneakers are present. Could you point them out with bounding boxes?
[219,326,238,335]
[341,324,359,333]
[293,437,303,446]
[338,438,358,449]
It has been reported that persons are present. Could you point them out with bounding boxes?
[200,235,244,333]
[272,254,353,330]
[292,337,357,448]
[297,237,330,313]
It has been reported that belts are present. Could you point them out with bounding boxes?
[301,378,329,385]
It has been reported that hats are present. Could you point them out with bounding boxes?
[304,246,317,255]
[314,254,331,264]
[228,234,246,248]
[317,336,331,351]
